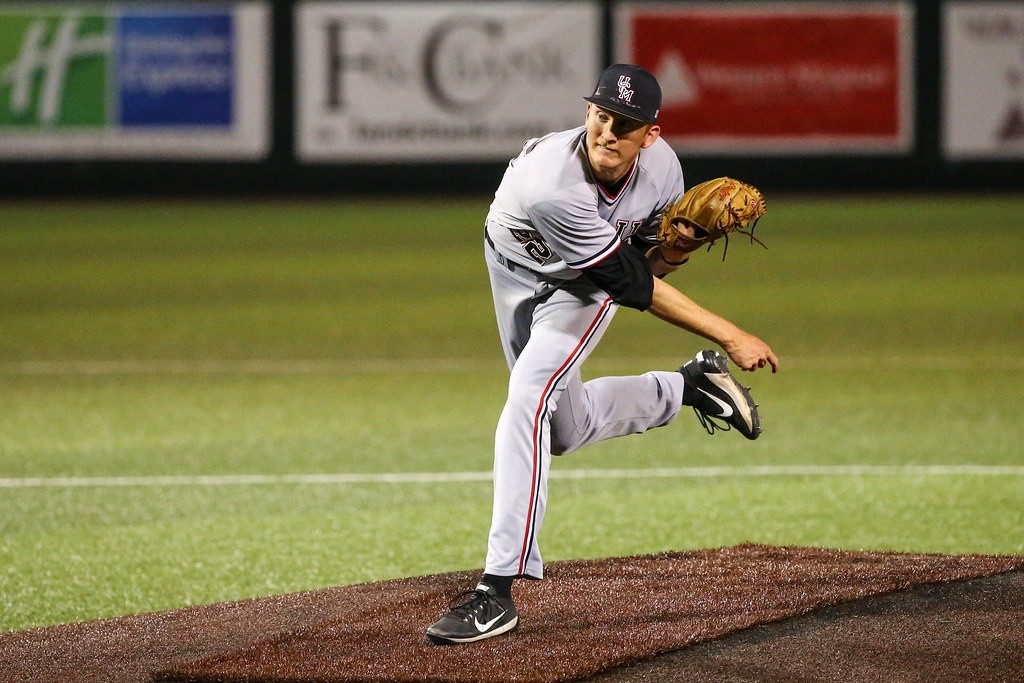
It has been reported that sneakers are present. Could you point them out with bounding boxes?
[426,581,519,644]
[680,350,762,440]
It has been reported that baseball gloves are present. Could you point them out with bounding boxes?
[635,177,769,268]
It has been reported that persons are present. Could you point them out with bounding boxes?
[426,64,779,644]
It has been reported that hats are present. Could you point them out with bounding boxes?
[583,63,662,123]
